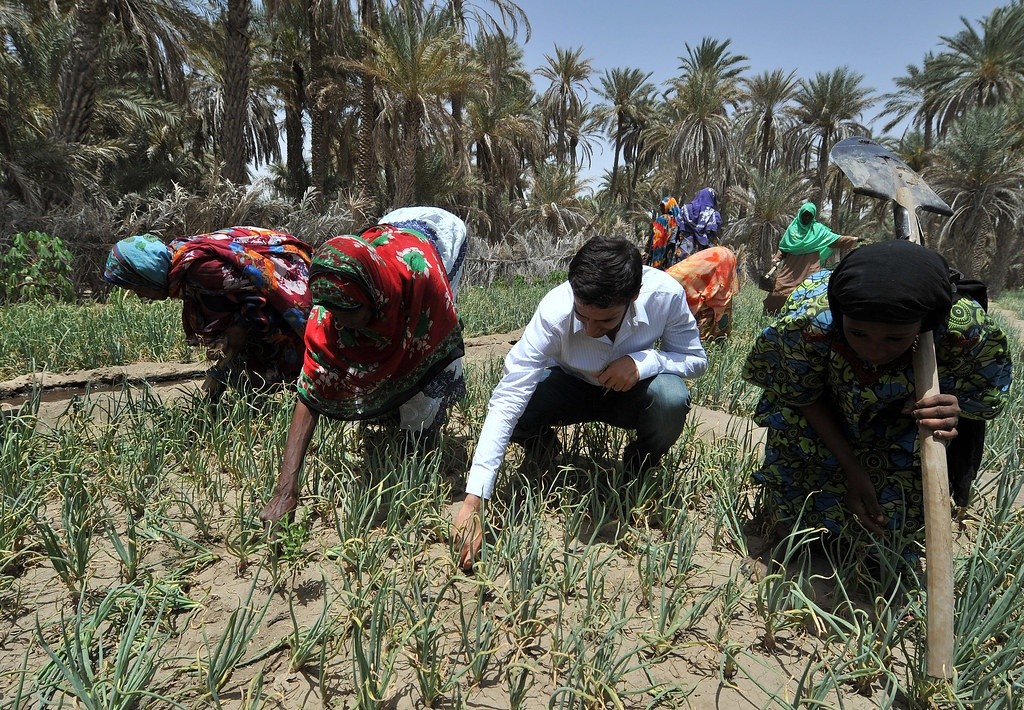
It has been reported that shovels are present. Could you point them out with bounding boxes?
[828,138,956,682]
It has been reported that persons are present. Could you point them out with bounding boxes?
[741,241,1014,602]
[644,188,740,345]
[763,203,865,318]
[104,226,319,421]
[450,236,708,570]
[259,207,468,558]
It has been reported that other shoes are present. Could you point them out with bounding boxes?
[624,451,640,483]
[519,440,561,471]
